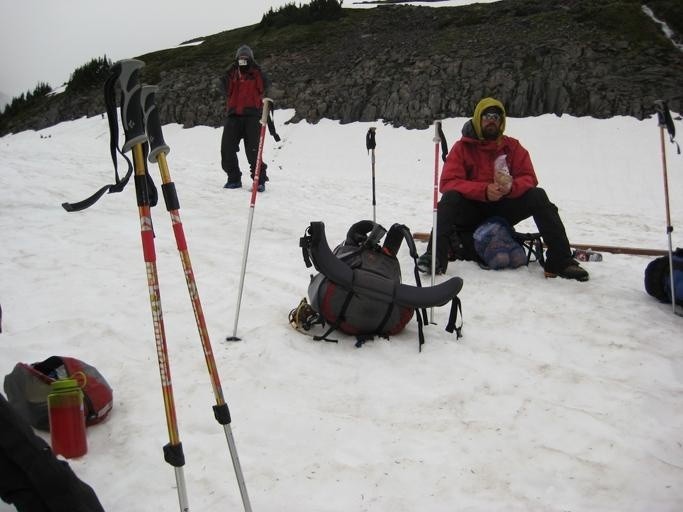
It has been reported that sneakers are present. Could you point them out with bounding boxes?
[256,182,265,193]
[416,251,447,275]
[543,258,590,282]
[222,181,242,189]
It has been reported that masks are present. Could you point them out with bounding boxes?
[238,59,249,67]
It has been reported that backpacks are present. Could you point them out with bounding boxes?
[298,218,464,339]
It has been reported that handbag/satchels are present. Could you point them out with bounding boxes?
[1,353,114,433]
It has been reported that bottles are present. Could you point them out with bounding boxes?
[45,369,88,459]
[570,249,602,262]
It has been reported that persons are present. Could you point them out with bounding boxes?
[416,97,591,281]
[209,44,270,192]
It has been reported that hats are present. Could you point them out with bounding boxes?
[234,44,255,63]
[471,96,507,141]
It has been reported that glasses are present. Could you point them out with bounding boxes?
[481,113,504,121]
